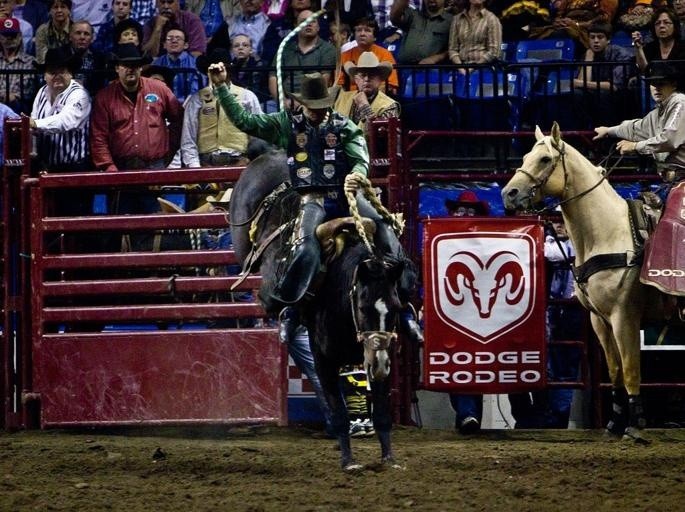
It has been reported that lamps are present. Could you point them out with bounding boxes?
[373,31,633,145]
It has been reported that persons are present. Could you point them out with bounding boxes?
[590,60,684,324]
[437,192,685,435]
[2,2,402,330]
[207,62,425,349]
[390,1,685,95]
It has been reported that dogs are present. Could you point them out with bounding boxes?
[228,148,406,471]
[501,120,685,442]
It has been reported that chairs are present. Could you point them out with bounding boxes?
[460,418,477,433]
[349,419,374,437]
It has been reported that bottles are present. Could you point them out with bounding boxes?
[106,42,153,69]
[207,188,233,205]
[285,73,340,108]
[639,60,675,83]
[345,51,393,78]
[0,18,20,34]
[31,46,84,72]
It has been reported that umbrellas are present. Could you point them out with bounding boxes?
[278,303,297,342]
